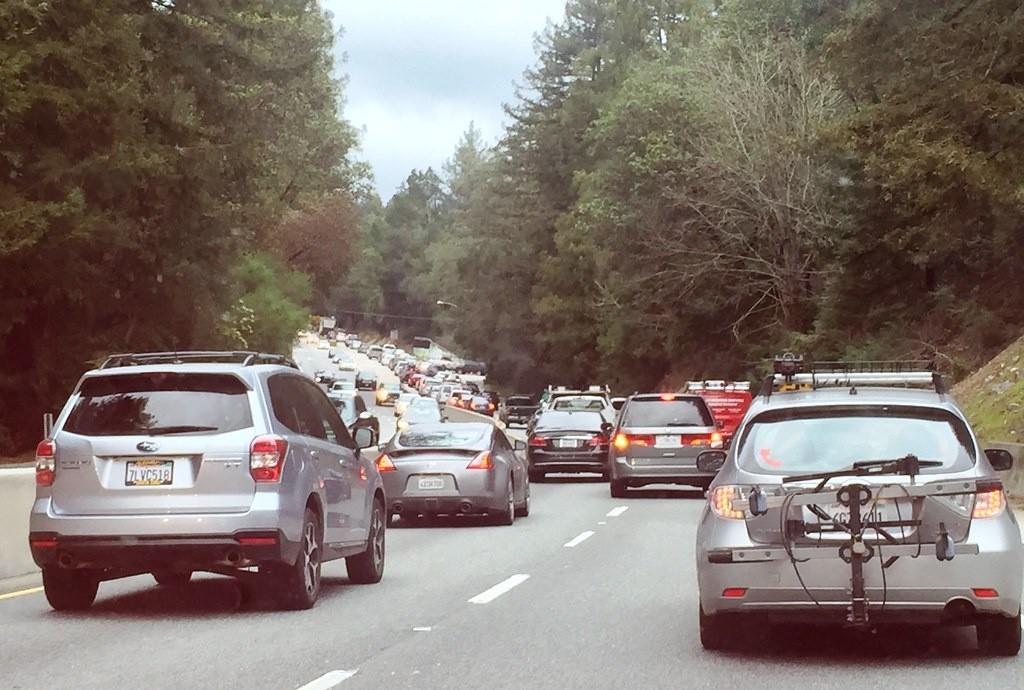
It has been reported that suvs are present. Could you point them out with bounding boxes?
[29,350,387,610]
[680,380,753,449]
[601,391,729,497]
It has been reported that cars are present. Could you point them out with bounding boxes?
[309,313,500,433]
[532,385,628,433]
[526,411,610,482]
[375,423,530,525]
[325,393,379,446]
[696,353,1024,655]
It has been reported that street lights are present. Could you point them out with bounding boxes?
[436,301,457,309]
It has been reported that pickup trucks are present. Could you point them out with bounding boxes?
[498,395,540,428]
